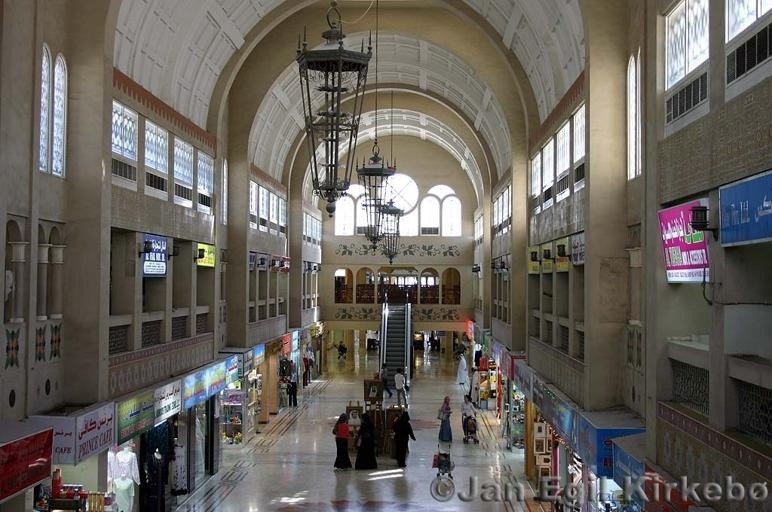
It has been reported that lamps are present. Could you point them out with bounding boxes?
[688,205,719,243]
[295,0,405,267]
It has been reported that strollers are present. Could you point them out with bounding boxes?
[436,441,453,484]
[461,412,480,445]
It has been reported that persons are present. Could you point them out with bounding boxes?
[348,410,362,424]
[144,448,167,484]
[354,412,379,471]
[115,447,141,486]
[437,395,454,442]
[392,411,416,468]
[469,366,480,407]
[286,372,298,408]
[337,341,345,360]
[431,329,436,352]
[107,451,116,481]
[460,395,479,432]
[468,367,475,397]
[455,355,467,386]
[112,474,136,512]
[394,368,408,408]
[380,363,393,398]
[332,412,353,469]
[454,335,459,352]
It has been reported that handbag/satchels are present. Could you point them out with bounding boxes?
[437,410,447,420]
